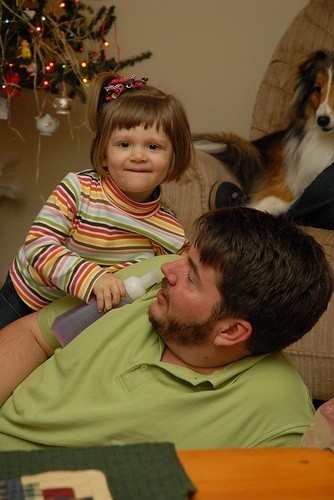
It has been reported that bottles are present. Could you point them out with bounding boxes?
[50,269,163,347]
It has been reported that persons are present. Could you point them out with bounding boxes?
[0,72,193,332]
[0,206,334,451]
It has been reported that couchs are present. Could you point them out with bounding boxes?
[159,0,334,407]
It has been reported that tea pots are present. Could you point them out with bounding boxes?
[35,113,60,136]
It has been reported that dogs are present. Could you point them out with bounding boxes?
[191,48,334,218]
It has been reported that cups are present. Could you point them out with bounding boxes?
[53,98,72,114]
